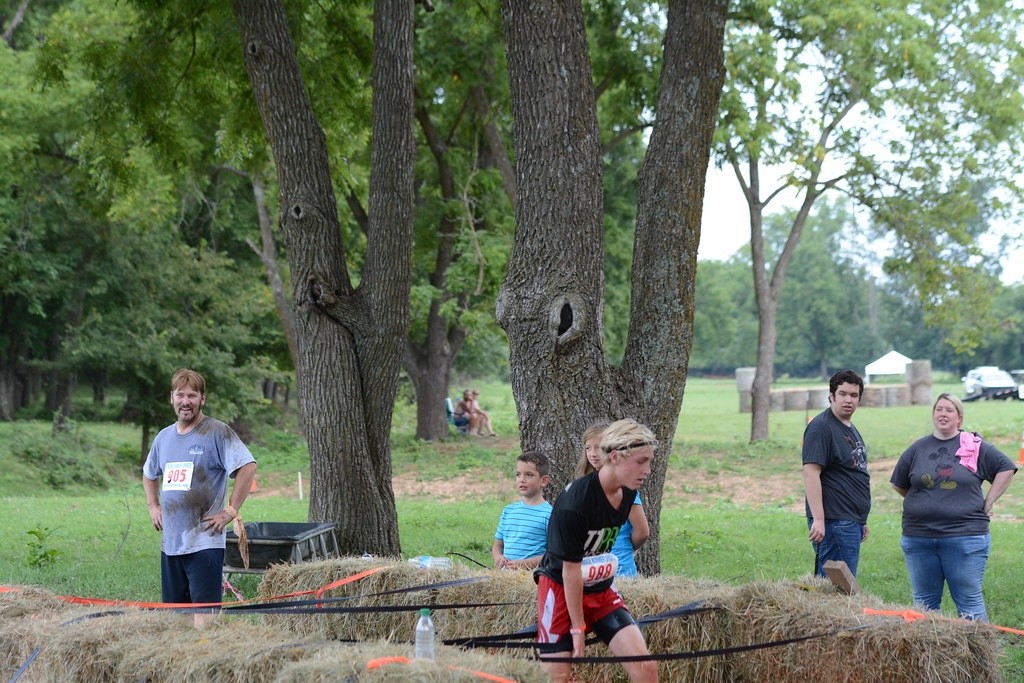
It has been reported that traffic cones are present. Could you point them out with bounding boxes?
[1015,439,1023,465]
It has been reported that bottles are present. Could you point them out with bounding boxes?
[408,556,448,570]
[415,609,434,662]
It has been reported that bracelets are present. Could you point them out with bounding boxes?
[569,622,587,635]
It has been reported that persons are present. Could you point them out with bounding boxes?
[492,450,554,571]
[890,392,1018,625]
[453,390,498,438]
[533,418,659,683]
[802,368,871,581]
[142,368,257,607]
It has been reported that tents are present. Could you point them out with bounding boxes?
[865,350,913,386]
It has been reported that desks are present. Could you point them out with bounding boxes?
[222,566,265,584]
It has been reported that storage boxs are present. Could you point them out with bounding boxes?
[224,521,339,567]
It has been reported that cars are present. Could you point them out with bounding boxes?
[969,366,1019,401]
[961,371,983,396]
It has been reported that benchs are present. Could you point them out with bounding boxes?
[444,398,470,433]
